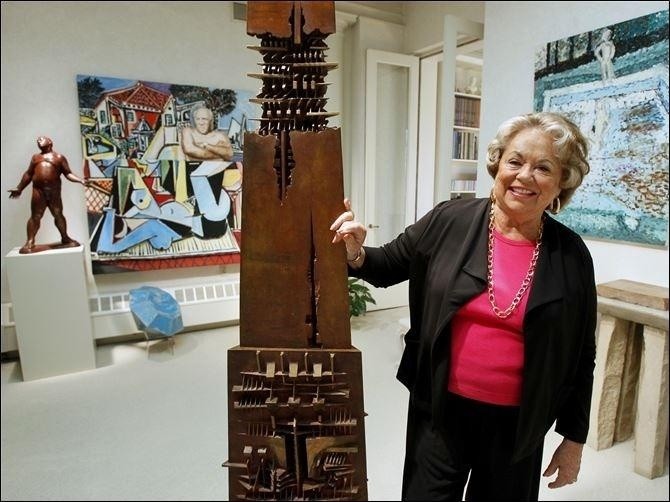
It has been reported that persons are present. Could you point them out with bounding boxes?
[595,28,616,84]
[181,102,234,164]
[329,112,597,500]
[7,136,79,252]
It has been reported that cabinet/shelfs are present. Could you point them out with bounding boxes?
[450,66,481,199]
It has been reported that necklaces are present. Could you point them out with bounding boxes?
[489,203,545,319]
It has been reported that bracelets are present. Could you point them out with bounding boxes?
[354,248,364,266]
